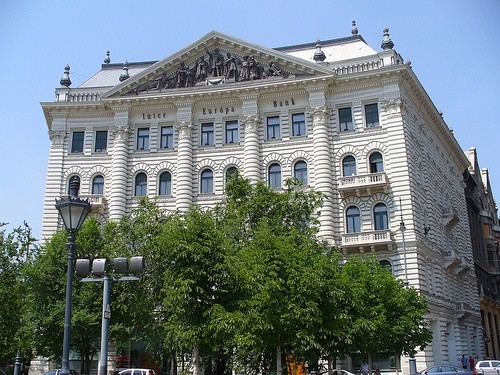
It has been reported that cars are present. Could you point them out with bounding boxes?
[43,370,77,374]
[416,363,477,375]
[320,370,357,375]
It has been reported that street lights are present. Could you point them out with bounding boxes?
[53,176,92,375]
[72,255,145,374]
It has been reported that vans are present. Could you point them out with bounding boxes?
[118,368,155,375]
[476,360,500,375]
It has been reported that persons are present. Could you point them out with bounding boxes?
[468,357,475,370]
[157,48,281,90]
[461,354,468,369]
[360,360,369,375]
[375,369,381,375]
